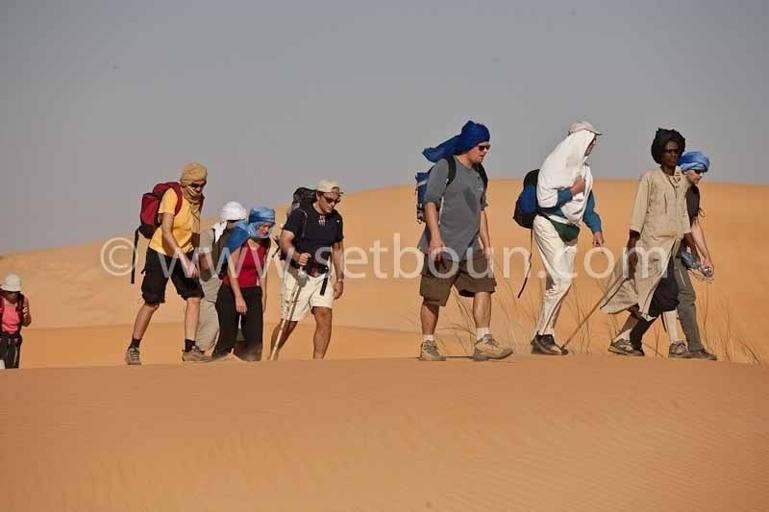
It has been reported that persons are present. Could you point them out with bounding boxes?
[416,120,514,361]
[125,163,211,364]
[211,206,276,362]
[633,152,716,359]
[531,120,604,354]
[608,128,701,357]
[0,274,32,369]
[196,201,250,354]
[266,179,344,360]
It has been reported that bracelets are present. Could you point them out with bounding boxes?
[337,278,344,283]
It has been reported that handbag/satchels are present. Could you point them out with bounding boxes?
[550,218,581,243]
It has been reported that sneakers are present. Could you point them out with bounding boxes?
[607,338,643,358]
[419,339,448,362]
[182,345,214,364]
[124,348,142,366]
[471,332,515,362]
[684,347,719,362]
[668,340,689,358]
[530,330,568,356]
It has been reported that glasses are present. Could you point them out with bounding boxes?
[477,144,491,151]
[321,195,341,205]
[688,168,705,175]
[188,182,207,189]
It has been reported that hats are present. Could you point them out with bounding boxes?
[0,273,21,294]
[567,120,603,139]
[314,180,345,194]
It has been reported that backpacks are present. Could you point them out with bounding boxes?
[277,186,317,261]
[512,166,540,229]
[414,154,456,223]
[139,179,183,239]
